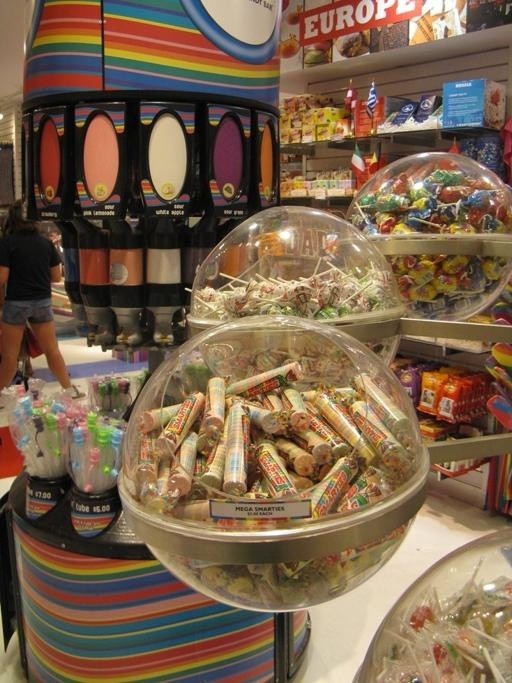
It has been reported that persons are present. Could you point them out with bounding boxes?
[0,199,86,409]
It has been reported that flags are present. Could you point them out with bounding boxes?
[342,82,378,178]
[439,145,460,169]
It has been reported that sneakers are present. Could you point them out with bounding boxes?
[64,385,87,401]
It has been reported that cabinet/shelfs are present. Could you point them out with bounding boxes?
[270,121,510,501]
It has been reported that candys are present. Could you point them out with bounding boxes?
[136,158,512,683]
